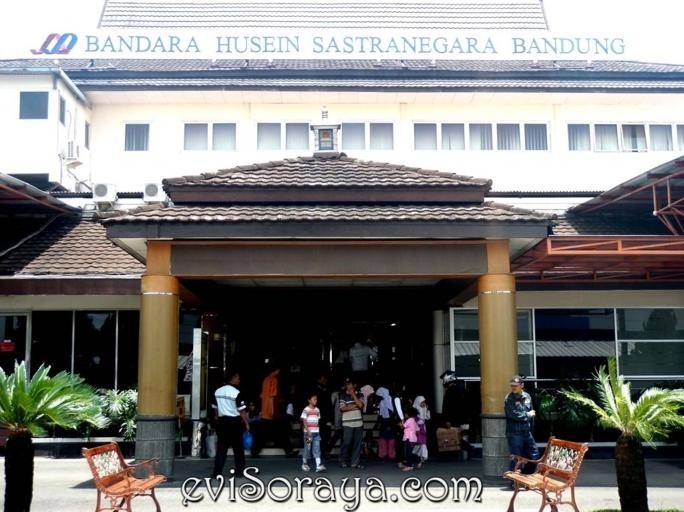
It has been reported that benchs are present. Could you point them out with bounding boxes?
[81,439,169,512]
[505,435,591,512]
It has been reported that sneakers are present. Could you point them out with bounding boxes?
[302,464,310,472]
[316,465,326,472]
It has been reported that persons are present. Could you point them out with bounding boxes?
[205,361,435,481]
[502,374,541,490]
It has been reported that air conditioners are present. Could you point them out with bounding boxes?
[141,181,168,206]
[91,182,120,205]
[68,139,86,164]
